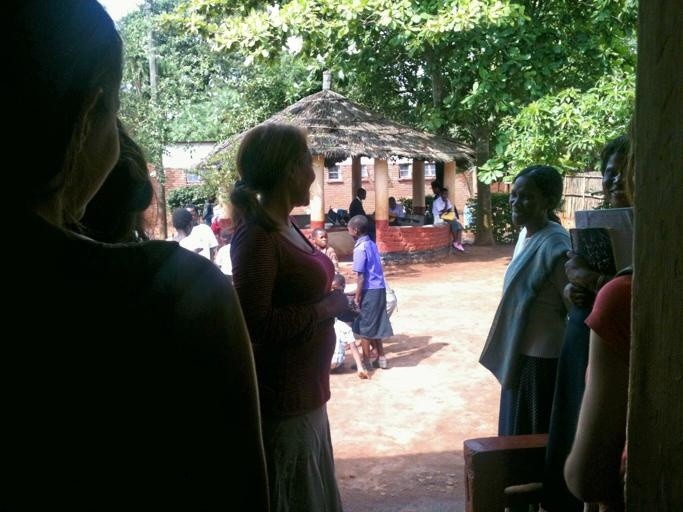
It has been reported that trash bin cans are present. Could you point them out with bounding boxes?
[463,204,474,228]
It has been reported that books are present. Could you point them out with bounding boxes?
[568,206,634,281]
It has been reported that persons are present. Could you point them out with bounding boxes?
[433,188,465,251]
[67,115,162,252]
[559,107,633,511]
[389,181,441,226]
[540,132,630,512]
[348,188,366,221]
[308,214,397,379]
[0,1,274,509]
[478,160,572,435]
[228,124,351,512]
[166,204,234,279]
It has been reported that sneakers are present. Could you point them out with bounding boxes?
[451,239,464,253]
